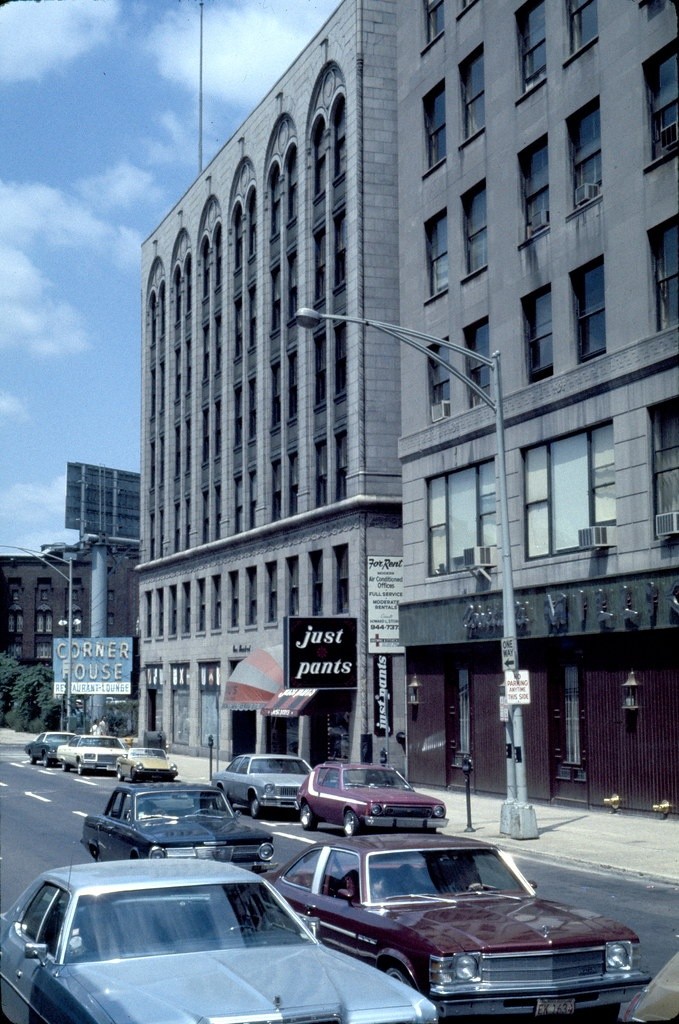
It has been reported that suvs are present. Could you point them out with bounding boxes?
[294,761,449,838]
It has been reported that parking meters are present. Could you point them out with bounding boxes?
[208,734,214,781]
[462,756,476,833]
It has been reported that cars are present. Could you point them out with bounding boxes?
[624,953,679,1024]
[56,735,129,776]
[256,834,652,1024]
[81,783,281,874]
[23,732,77,768]
[212,753,314,821]
[0,857,438,1024]
[116,747,179,782]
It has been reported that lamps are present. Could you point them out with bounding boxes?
[408,674,421,706]
[621,669,640,710]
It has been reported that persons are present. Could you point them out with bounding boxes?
[444,856,483,892]
[89,715,109,736]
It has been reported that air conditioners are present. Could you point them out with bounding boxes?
[660,121,678,149]
[531,210,551,231]
[655,513,679,536]
[430,400,449,422]
[464,547,496,566]
[575,182,599,205]
[578,526,608,550]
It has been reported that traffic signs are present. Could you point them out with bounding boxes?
[501,636,516,670]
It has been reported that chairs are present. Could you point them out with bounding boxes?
[139,797,160,817]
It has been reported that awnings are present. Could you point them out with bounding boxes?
[261,687,357,718]
[221,643,284,711]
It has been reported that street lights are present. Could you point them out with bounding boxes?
[294,306,539,840]
